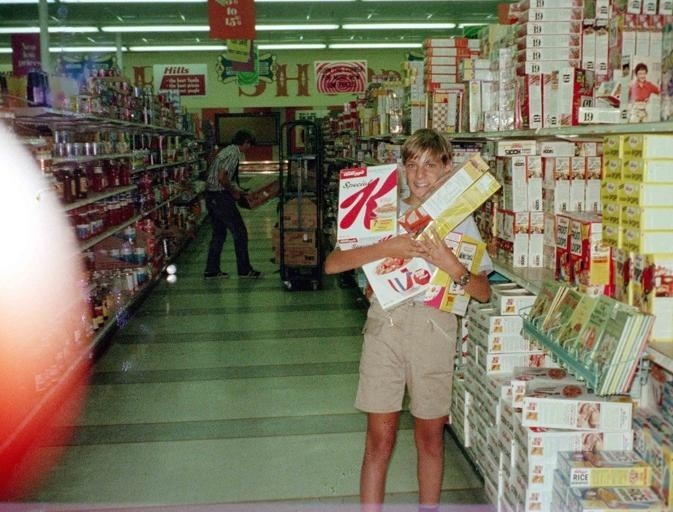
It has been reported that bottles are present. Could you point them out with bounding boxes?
[22,69,138,330]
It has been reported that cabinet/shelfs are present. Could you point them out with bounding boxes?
[279,2,673,512]
[0,101,217,456]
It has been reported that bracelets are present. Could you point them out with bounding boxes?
[453,267,471,289]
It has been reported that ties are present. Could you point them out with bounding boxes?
[230,157,251,193]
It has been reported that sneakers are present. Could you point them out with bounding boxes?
[203,271,230,280]
[237,269,265,279]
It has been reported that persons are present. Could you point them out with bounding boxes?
[626,62,662,124]
[203,128,263,278]
[321,126,494,512]
